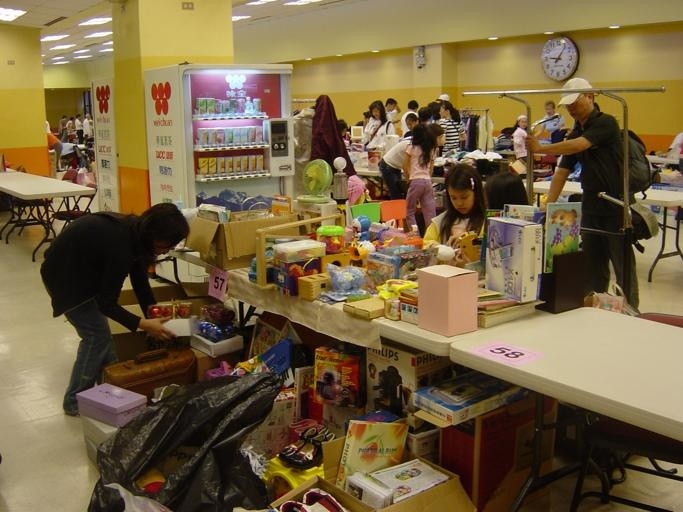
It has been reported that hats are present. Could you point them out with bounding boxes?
[557,78,592,108]
[436,94,449,101]
[517,115,527,121]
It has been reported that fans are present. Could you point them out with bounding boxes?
[299,159,332,204]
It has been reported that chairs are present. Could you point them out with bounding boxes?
[52,184,97,248]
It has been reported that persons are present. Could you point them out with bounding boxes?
[524,78,639,315]
[337,94,565,265]
[40,203,191,416]
[45,113,93,172]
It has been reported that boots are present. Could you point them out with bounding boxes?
[288,419,317,444]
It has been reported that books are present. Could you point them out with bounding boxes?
[335,421,409,491]
[477,288,536,329]
[343,297,385,320]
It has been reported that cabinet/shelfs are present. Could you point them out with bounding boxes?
[143,62,292,203]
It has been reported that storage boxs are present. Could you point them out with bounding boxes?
[239,390,298,461]
[185,208,302,272]
[313,346,362,405]
[269,475,376,512]
[484,217,543,303]
[75,415,154,467]
[76,383,156,428]
[365,339,454,416]
[439,395,555,508]
[416,264,479,337]
[322,433,474,508]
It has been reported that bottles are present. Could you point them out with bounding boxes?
[244,97,254,115]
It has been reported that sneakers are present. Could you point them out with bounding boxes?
[280,500,310,512]
[65,409,79,416]
[302,488,346,512]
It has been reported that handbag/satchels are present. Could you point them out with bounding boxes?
[584,284,640,317]
[103,348,196,399]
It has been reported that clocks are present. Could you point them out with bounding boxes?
[540,36,580,83]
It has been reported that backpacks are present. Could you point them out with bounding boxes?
[621,128,651,200]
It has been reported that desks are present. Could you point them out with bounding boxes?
[352,168,390,200]
[162,236,557,359]
[531,181,682,282]
[0,166,96,262]
[451,306,682,507]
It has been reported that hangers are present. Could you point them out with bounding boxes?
[455,109,490,116]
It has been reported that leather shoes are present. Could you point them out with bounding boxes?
[288,433,336,469]
[279,424,328,459]
